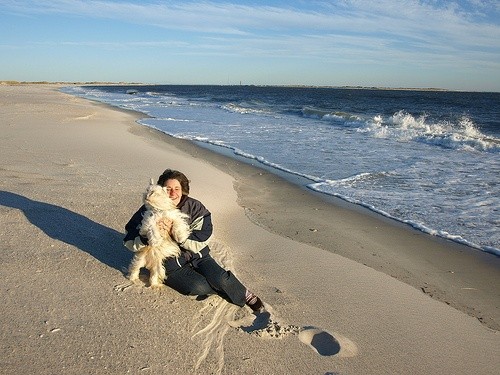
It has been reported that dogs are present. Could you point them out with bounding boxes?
[128,178,191,288]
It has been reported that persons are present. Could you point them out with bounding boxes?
[123,169,264,311]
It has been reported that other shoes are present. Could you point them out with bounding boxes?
[245,295,262,309]
